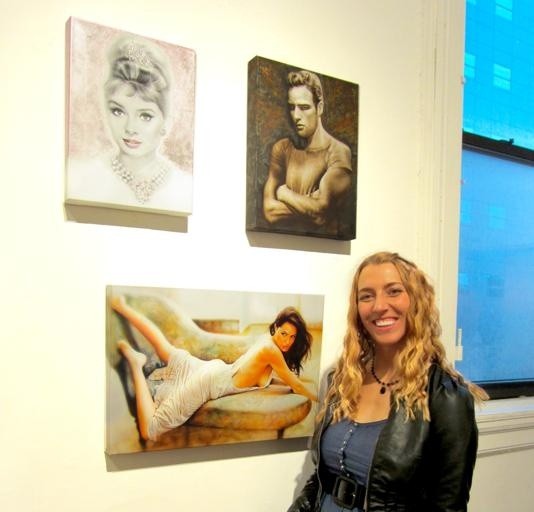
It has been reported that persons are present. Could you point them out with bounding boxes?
[285,252,492,512]
[77,36,185,208]
[262,70,353,236]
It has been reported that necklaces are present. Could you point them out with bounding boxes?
[370,348,401,396]
[112,156,169,204]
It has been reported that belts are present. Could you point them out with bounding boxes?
[322,477,368,511]
